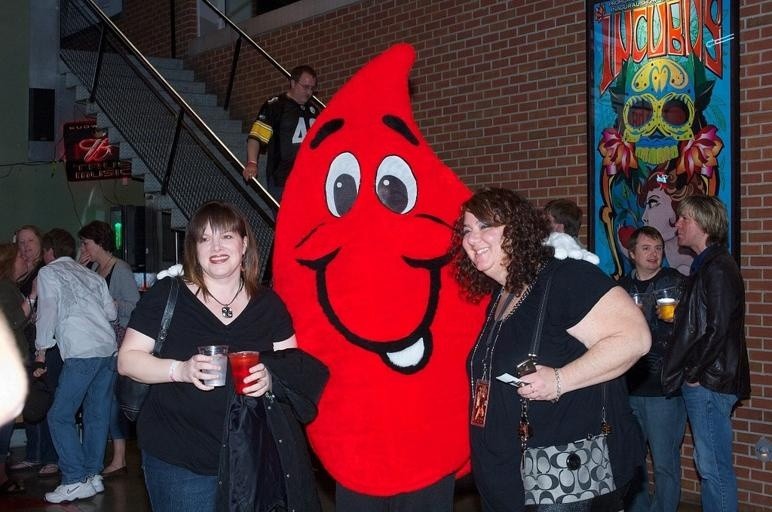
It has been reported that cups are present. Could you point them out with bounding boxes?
[629,293,651,323]
[230,350,260,396]
[197,344,228,387]
[653,286,678,320]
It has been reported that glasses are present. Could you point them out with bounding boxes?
[297,82,316,89]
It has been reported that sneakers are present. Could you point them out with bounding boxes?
[89,475,105,494]
[11,461,37,469]
[38,463,59,476]
[44,478,96,503]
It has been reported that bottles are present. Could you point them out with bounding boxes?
[75,162,124,178]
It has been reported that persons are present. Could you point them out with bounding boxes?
[0,220,140,504]
[243,66,320,217]
[448,187,652,512]
[615,226,689,512]
[117,200,298,512]
[656,195,751,512]
[544,199,587,247]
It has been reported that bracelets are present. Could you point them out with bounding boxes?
[550,366,561,406]
[169,361,181,383]
[245,161,258,166]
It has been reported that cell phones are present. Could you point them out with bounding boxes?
[516,359,538,378]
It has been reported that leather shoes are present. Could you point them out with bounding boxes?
[101,466,127,478]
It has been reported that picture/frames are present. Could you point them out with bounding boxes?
[582,0,742,286]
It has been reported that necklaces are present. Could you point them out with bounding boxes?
[469,260,547,399]
[198,279,244,319]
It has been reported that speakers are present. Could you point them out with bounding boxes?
[29,88,55,141]
